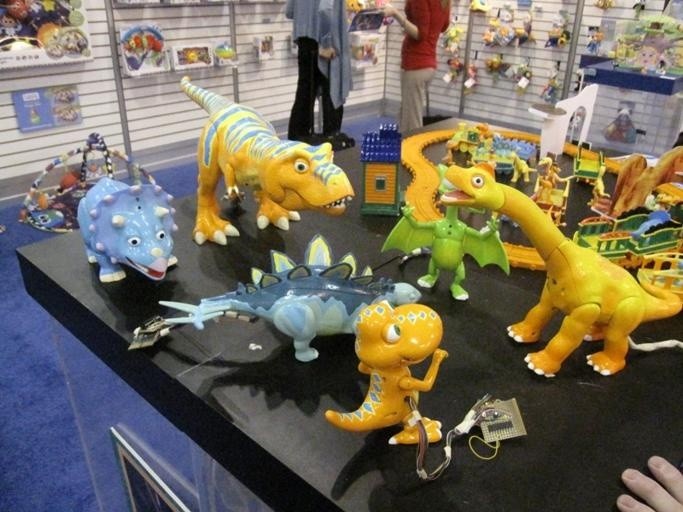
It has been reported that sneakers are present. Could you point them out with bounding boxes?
[298,134,356,152]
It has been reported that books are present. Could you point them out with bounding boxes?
[9,82,83,133]
[0,0,95,72]
[347,7,384,31]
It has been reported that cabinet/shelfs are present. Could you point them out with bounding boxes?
[577,51,683,160]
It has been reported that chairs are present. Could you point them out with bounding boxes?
[527,81,600,154]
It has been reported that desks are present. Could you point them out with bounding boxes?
[16,116,683,512]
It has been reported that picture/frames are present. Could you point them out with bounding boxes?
[170,44,214,71]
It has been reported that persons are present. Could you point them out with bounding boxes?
[284,1,357,155]
[377,1,452,138]
[611,446,682,510]
